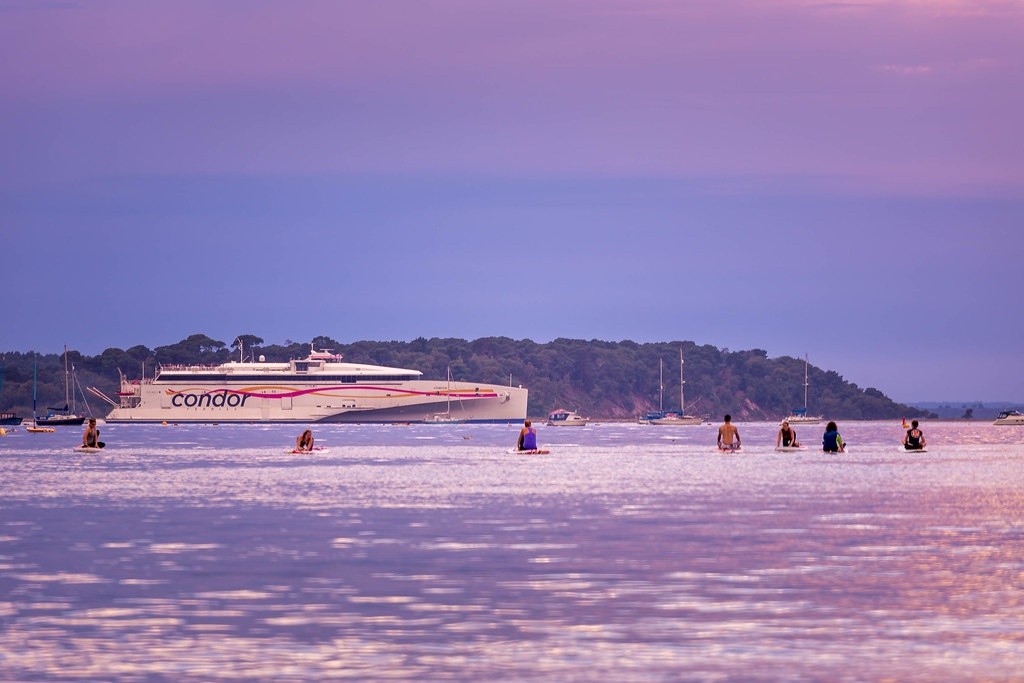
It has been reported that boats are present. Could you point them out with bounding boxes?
[547,408,588,426]
[897,445,927,453]
[46,343,106,424]
[0,412,23,425]
[26,426,55,432]
[35,415,86,425]
[992,410,1024,425]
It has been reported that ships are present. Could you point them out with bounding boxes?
[85,334,529,424]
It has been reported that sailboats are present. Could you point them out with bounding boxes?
[778,353,824,425]
[648,348,703,425]
[637,357,663,425]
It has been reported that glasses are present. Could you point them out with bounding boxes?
[91,423,96,426]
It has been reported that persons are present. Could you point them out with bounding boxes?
[717,414,742,449]
[904,419,926,449]
[132,377,140,385]
[296,429,314,451]
[82,417,105,449]
[518,420,537,450]
[822,421,845,452]
[777,422,800,447]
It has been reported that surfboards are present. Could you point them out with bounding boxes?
[897,444,928,453]
[507,448,550,455]
[710,446,743,454]
[291,447,331,455]
[825,444,848,456]
[75,444,104,453]
[777,445,808,453]
